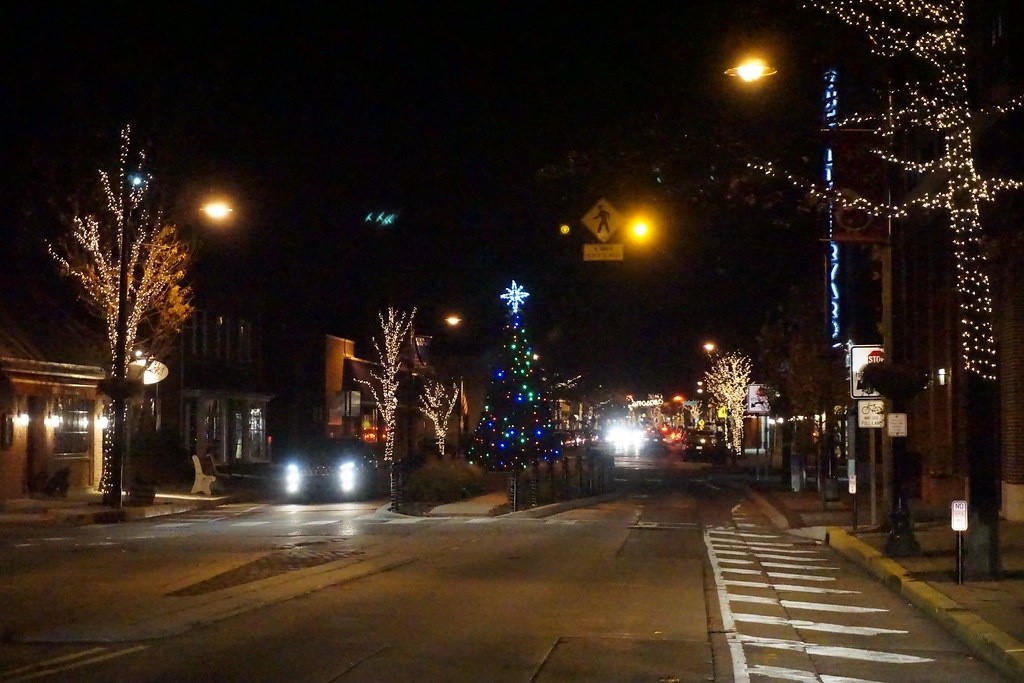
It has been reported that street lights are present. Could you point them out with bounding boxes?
[96,202,234,526]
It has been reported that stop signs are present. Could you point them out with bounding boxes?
[757,388,766,397]
[867,350,887,366]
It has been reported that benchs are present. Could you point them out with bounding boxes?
[188,453,232,496]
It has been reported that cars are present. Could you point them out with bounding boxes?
[288,438,383,500]
